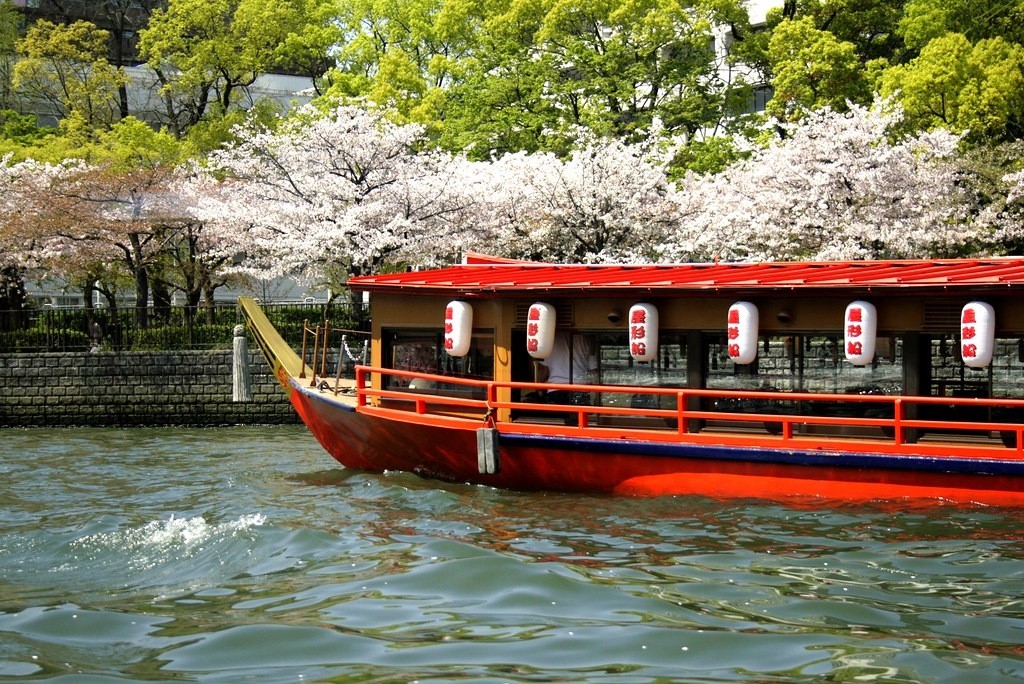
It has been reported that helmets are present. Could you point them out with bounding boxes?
[408,378,436,393]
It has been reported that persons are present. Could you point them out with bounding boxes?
[537,331,598,417]
[88,314,105,344]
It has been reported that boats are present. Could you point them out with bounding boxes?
[231,250,1023,507]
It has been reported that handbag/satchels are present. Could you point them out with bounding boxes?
[570,392,591,405]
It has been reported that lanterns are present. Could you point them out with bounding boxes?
[443,296,473,359]
[960,302,995,371]
[628,302,658,364]
[844,300,877,368]
[727,302,758,365]
[526,300,557,362]
